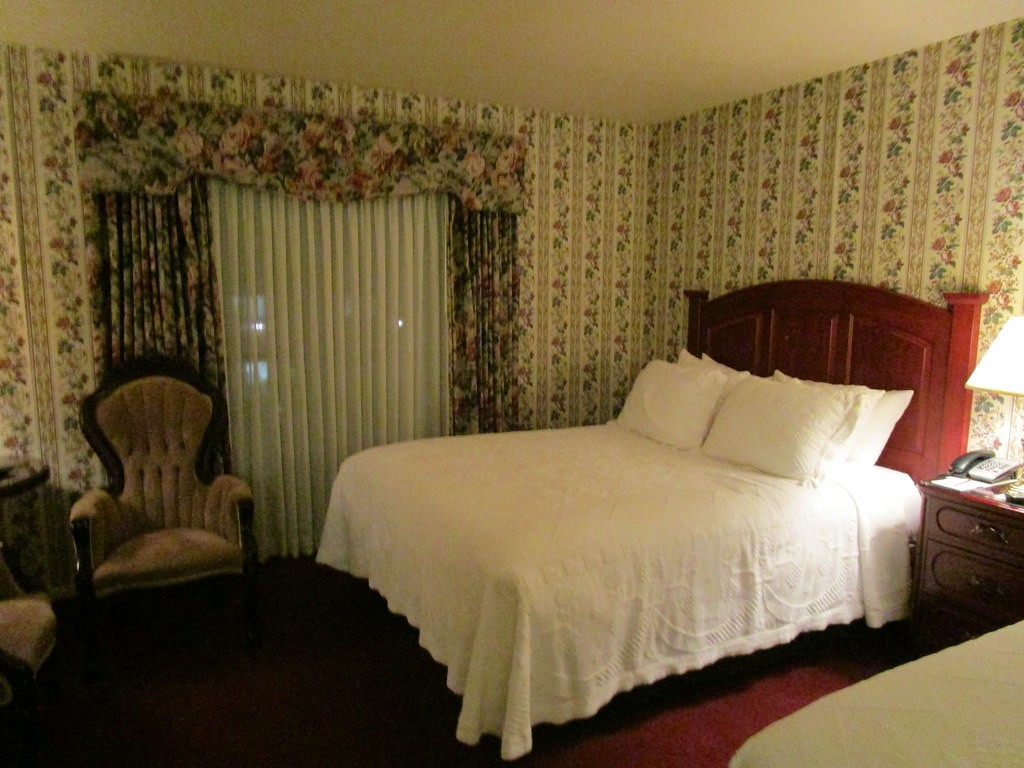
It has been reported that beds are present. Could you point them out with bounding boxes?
[313,279,990,759]
[728,620,1024,768]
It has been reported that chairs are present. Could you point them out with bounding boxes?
[0,597,57,768]
[68,366,265,684]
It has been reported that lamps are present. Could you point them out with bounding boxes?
[964,315,1024,505]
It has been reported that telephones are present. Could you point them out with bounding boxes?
[949,447,1021,484]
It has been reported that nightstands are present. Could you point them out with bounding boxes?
[905,474,1024,656]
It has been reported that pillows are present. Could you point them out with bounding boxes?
[617,348,914,488]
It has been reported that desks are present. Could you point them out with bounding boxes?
[0,462,51,596]
